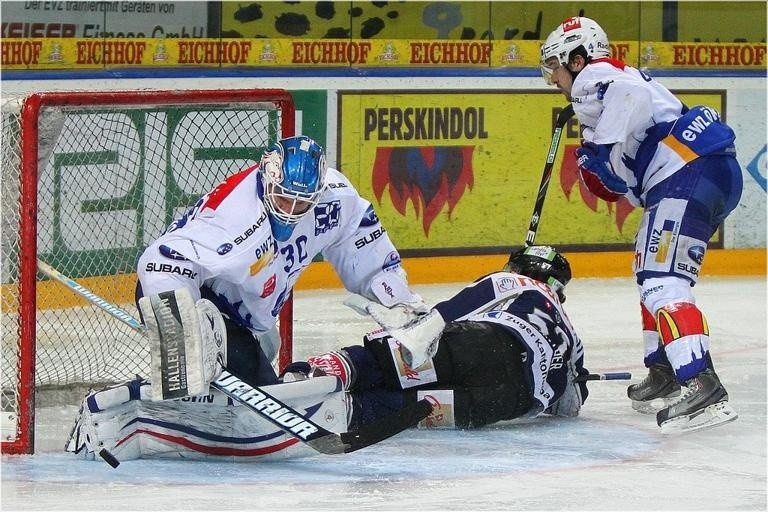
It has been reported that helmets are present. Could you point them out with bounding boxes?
[257,135,329,203]
[504,243,574,301]
[540,15,614,68]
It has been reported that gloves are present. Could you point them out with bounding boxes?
[574,141,628,202]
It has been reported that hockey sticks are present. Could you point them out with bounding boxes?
[35,257,431,456]
[525,104,575,248]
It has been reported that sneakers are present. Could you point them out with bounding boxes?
[626,364,682,402]
[656,368,729,427]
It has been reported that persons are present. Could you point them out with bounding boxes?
[540,16,743,427]
[134,134,448,386]
[279,244,590,429]
